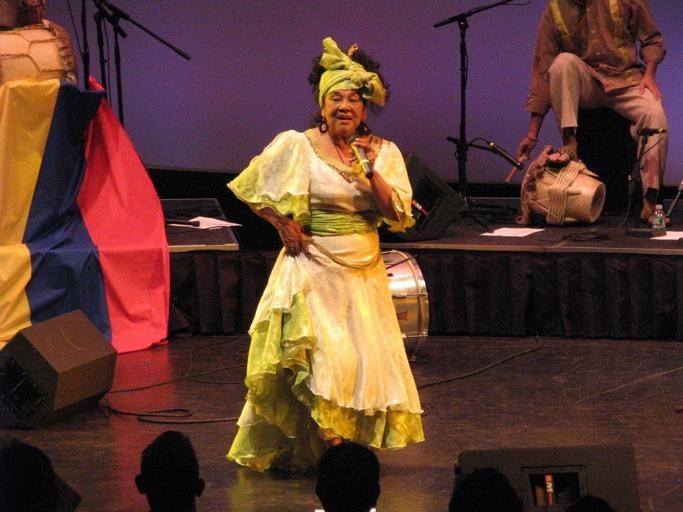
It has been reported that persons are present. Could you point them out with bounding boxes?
[514,0,671,228]
[132,431,205,511]
[446,467,523,511]
[315,440,381,511]
[224,49,426,473]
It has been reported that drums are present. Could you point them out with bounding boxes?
[516,146,607,226]
[381,250,429,361]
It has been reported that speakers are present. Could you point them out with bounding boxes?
[453,443,642,512]
[0,309,117,430]
[378,151,466,242]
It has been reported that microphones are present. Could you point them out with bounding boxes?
[637,128,667,136]
[348,133,373,179]
[486,140,523,170]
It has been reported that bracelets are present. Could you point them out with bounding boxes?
[526,136,536,142]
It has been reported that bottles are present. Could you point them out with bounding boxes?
[652,205,666,237]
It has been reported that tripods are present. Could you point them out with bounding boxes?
[433,1,518,234]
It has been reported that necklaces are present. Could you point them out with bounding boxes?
[327,130,352,168]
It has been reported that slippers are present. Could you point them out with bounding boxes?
[639,207,669,227]
[547,147,575,165]
